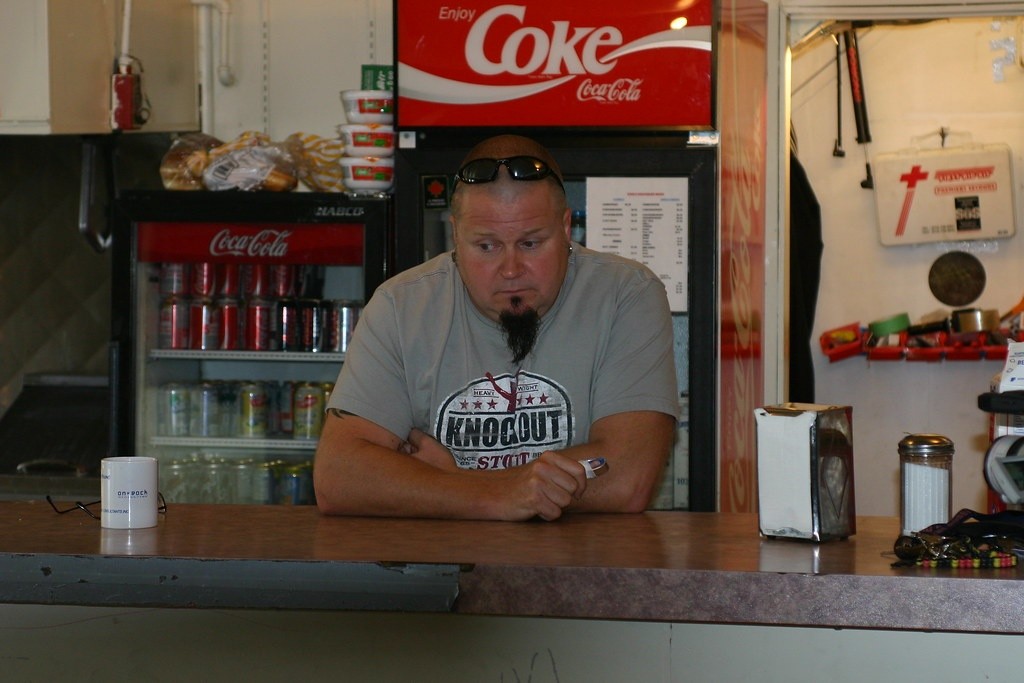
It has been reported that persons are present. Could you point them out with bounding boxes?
[313,135,681,522]
[787,120,824,405]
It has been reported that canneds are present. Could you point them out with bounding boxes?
[159,455,317,507]
[157,377,334,441]
[159,260,360,355]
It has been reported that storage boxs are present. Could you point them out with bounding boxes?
[752,402,856,539]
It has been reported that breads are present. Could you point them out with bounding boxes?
[159,131,298,191]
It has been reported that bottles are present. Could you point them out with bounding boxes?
[897,434,955,539]
[655,391,688,509]
[571,211,586,243]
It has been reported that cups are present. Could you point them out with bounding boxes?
[100,457,158,530]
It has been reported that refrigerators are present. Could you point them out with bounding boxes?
[392,0,767,515]
[111,192,386,505]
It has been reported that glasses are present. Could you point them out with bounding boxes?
[45,492,168,520]
[448,154,566,207]
